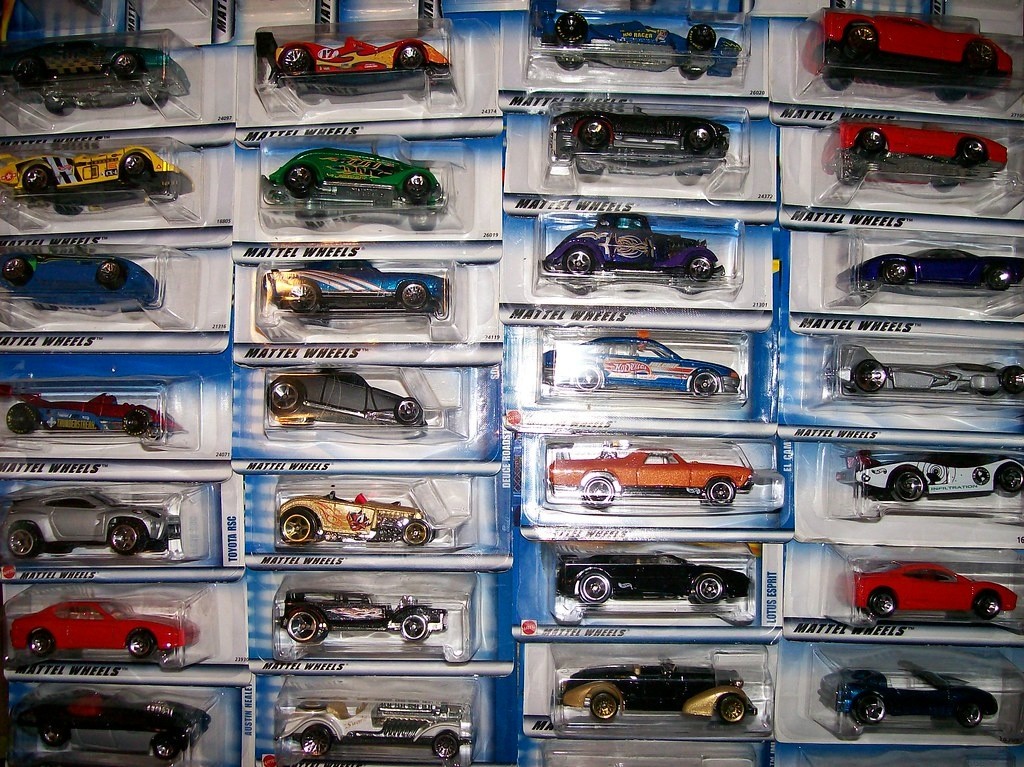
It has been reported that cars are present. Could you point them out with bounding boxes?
[838,559,1018,620]
[2,47,189,114]
[550,448,753,507]
[546,214,719,294]
[541,331,741,400]
[816,118,1007,192]
[804,10,1014,101]
[0,250,156,315]
[14,598,211,759]
[273,489,472,758]
[259,149,450,430]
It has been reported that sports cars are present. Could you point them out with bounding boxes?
[2,488,185,556]
[0,144,193,216]
[818,660,996,729]
[538,10,741,183]
[835,249,1023,502]
[556,550,756,604]
[562,662,761,724]
[0,381,186,444]
[255,28,457,96]
